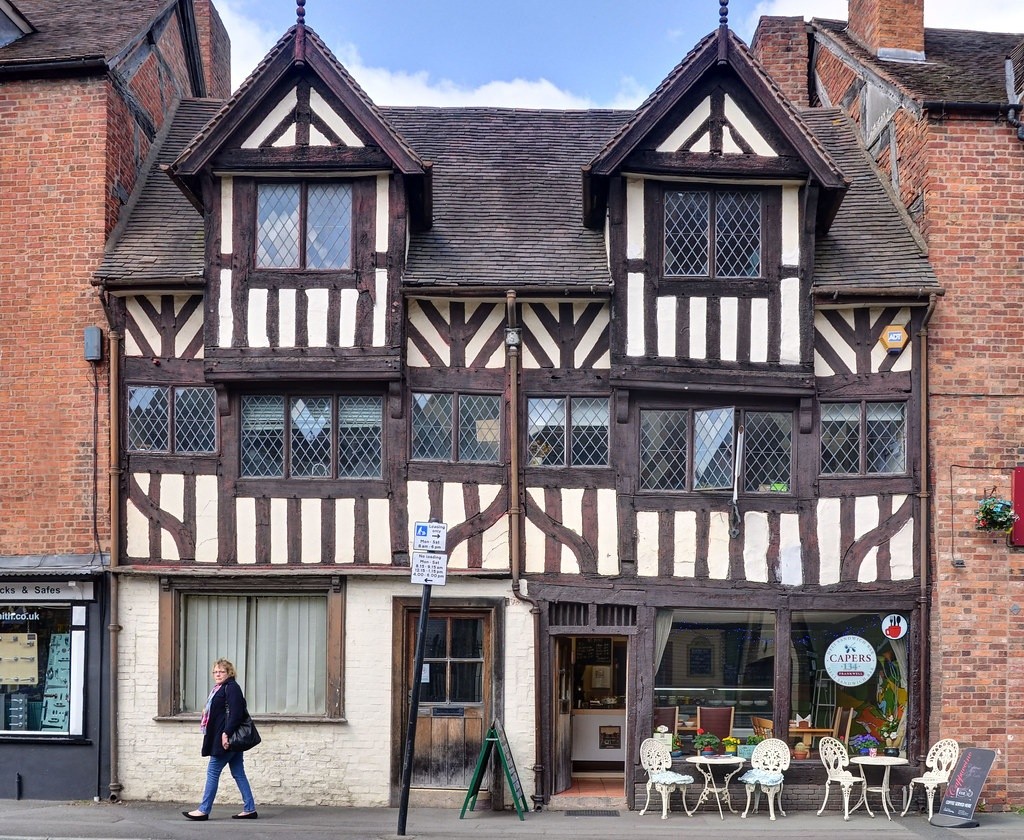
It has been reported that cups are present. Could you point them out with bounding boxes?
[869,748,877,757]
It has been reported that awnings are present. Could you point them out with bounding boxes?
[0,554,111,575]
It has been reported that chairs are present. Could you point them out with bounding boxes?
[737,738,791,821]
[817,736,875,821]
[638,738,693,819]
[697,706,734,756]
[901,739,959,822]
[811,706,853,758]
[653,706,679,739]
[751,715,794,757]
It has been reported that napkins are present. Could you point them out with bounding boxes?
[796,714,811,728]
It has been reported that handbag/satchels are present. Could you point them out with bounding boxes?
[224,709,262,753]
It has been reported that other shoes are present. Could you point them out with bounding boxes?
[182,811,209,820]
[231,811,258,819]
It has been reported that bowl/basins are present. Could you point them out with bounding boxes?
[723,701,737,705]
[678,723,683,726]
[739,701,753,705]
[754,701,768,705]
[708,700,722,705]
[685,722,695,727]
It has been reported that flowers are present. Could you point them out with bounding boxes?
[974,486,1021,533]
[691,729,720,751]
[878,715,900,741]
[721,736,741,747]
[840,735,844,743]
[849,733,880,750]
[528,440,551,457]
[671,730,684,752]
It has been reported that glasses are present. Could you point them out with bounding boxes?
[212,670,227,674]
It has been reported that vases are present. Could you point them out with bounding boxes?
[532,458,542,465]
[726,745,736,752]
[703,747,713,751]
[861,748,870,755]
[671,751,682,757]
[886,737,892,747]
[884,748,899,758]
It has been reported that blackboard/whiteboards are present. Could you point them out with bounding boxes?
[939,747,996,820]
[490,719,524,801]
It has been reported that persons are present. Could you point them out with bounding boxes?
[182,659,258,821]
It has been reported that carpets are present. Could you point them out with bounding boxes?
[564,810,620,817]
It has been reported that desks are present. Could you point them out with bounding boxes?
[850,756,909,821]
[789,728,833,751]
[677,724,697,744]
[686,755,747,820]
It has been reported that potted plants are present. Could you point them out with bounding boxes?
[745,735,764,746]
[793,742,807,760]
